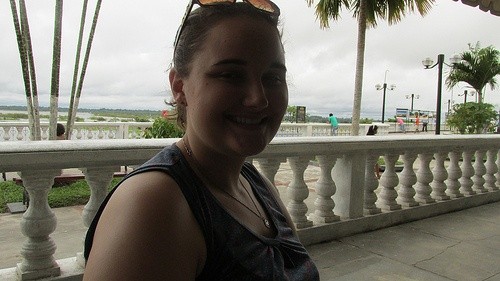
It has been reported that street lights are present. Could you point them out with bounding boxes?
[374,83,396,123]
[443,98,455,111]
[457,90,475,103]
[404,93,420,113]
[421,53,462,136]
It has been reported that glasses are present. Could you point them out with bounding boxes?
[171,0,280,67]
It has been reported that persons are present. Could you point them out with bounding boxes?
[394,115,428,133]
[329,113,339,135]
[83,0,319,281]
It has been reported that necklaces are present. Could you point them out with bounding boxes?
[183,139,270,228]
